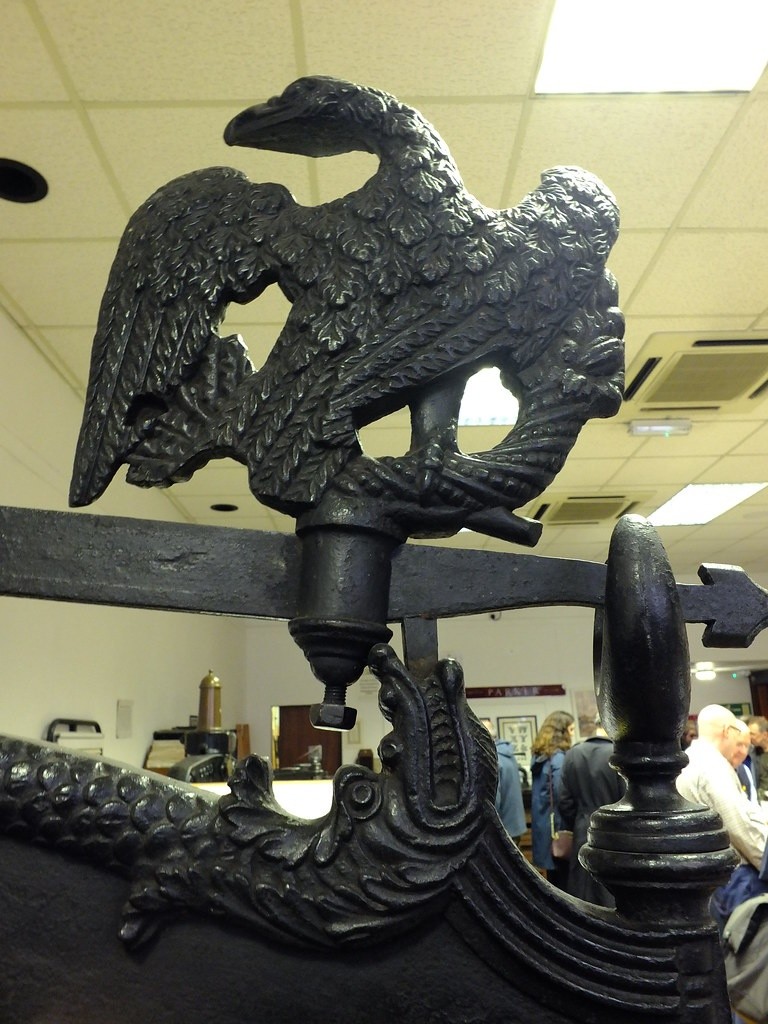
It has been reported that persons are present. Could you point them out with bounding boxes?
[680,720,698,752]
[675,704,768,1023]
[530,710,576,885]
[480,720,528,848]
[748,715,767,803]
[729,718,762,808]
[557,710,627,909]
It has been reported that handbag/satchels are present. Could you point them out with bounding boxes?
[725,892,768,1024]
[552,830,574,857]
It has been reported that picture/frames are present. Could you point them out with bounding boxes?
[496,715,538,786]
